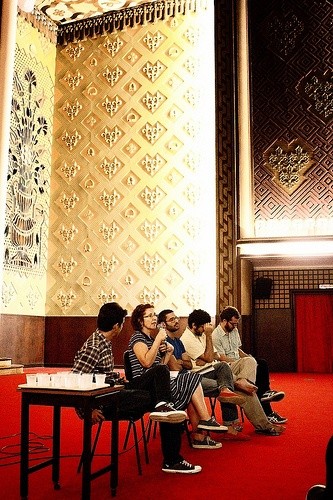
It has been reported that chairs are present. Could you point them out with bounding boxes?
[77,351,245,476]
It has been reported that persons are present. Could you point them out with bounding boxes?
[210,306,289,424]
[179,310,286,435]
[158,310,245,431]
[127,304,229,448]
[71,301,203,474]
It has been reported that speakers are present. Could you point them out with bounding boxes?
[255,278,272,299]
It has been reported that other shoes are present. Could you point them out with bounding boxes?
[223,432,250,441]
[255,423,286,436]
[217,393,246,405]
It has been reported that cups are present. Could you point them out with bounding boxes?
[26,374,37,386]
[37,371,93,389]
[95,374,106,387]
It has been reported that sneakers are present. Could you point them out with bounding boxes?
[192,436,222,449]
[162,457,202,474]
[148,400,186,424]
[261,390,285,403]
[266,411,288,423]
[197,416,228,433]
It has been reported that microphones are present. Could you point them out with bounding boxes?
[156,324,166,345]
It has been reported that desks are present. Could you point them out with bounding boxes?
[15,384,124,500]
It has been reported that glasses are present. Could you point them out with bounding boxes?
[165,316,178,322]
[142,314,157,318]
[228,321,238,326]
